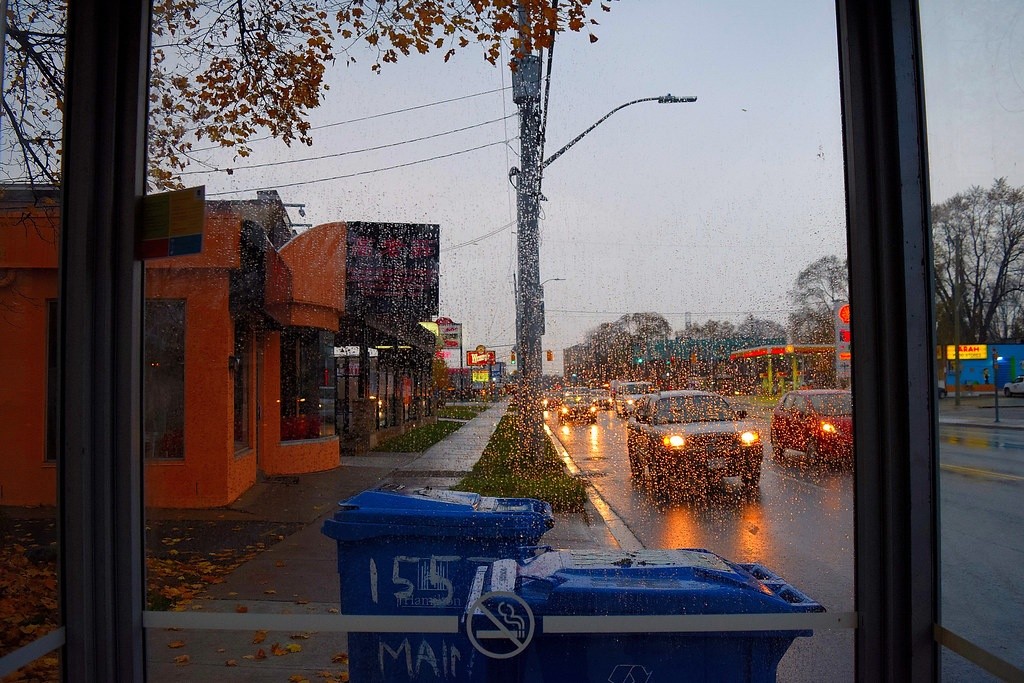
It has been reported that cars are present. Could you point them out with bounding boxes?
[537,381,610,431]
[936,386,947,400]
[1003,374,1024,398]
[771,390,857,470]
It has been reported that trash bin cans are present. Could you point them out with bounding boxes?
[318,483,557,682]
[510,547,828,683]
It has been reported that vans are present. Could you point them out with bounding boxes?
[614,381,655,422]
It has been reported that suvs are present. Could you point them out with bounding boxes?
[630,389,765,501]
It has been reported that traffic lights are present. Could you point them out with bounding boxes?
[511,354,516,363]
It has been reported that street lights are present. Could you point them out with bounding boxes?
[515,0,700,466]
